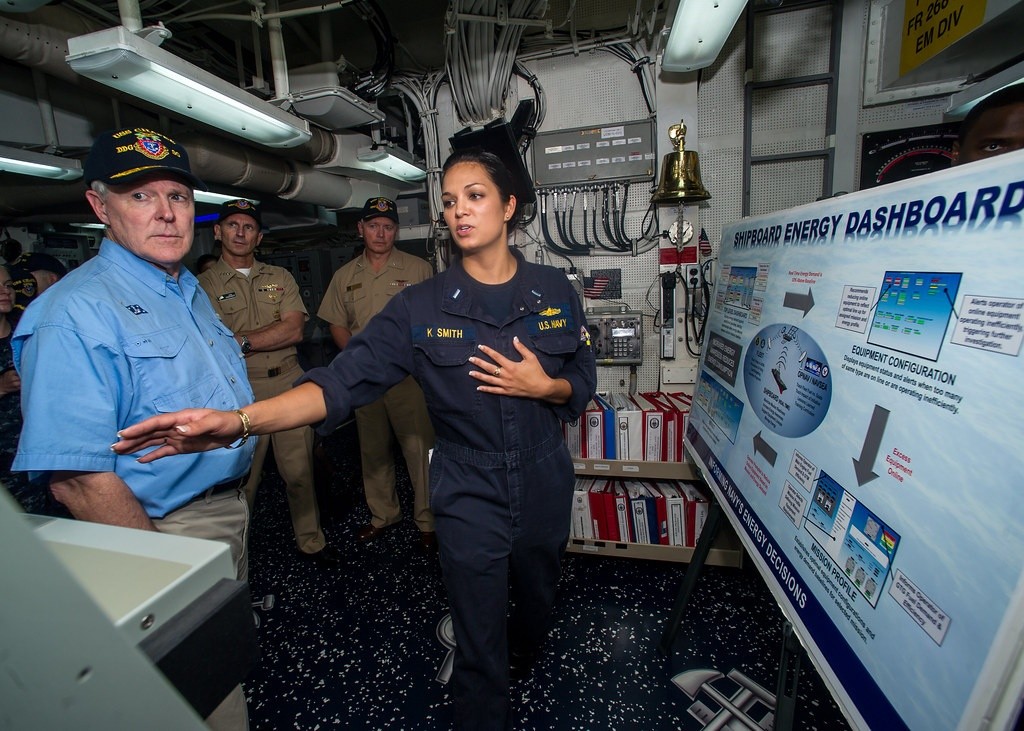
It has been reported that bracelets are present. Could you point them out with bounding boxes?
[227,410,250,450]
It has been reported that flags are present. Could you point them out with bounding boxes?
[584,277,609,297]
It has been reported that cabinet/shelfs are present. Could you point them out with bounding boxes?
[562,457,744,570]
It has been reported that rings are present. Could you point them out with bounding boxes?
[493,367,500,376]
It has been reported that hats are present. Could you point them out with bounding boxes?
[10,268,38,310]
[219,199,262,230]
[11,252,67,276]
[83,125,209,186]
[359,197,399,225]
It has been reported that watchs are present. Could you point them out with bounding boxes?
[242,336,251,354]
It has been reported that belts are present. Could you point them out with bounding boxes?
[193,466,251,499]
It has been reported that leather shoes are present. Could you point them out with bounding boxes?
[357,520,401,544]
[418,528,436,549]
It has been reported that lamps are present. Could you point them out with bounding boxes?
[191,184,261,207]
[659,0,747,74]
[69,222,106,230]
[944,61,1024,115]
[355,115,432,184]
[64,0,313,151]
[0,141,84,183]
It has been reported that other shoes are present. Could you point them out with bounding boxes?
[507,639,533,681]
[301,547,343,568]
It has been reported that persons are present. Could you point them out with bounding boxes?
[0,253,66,514]
[111,148,596,729]
[196,254,217,274]
[196,200,327,570]
[316,198,435,543]
[10,127,255,731]
